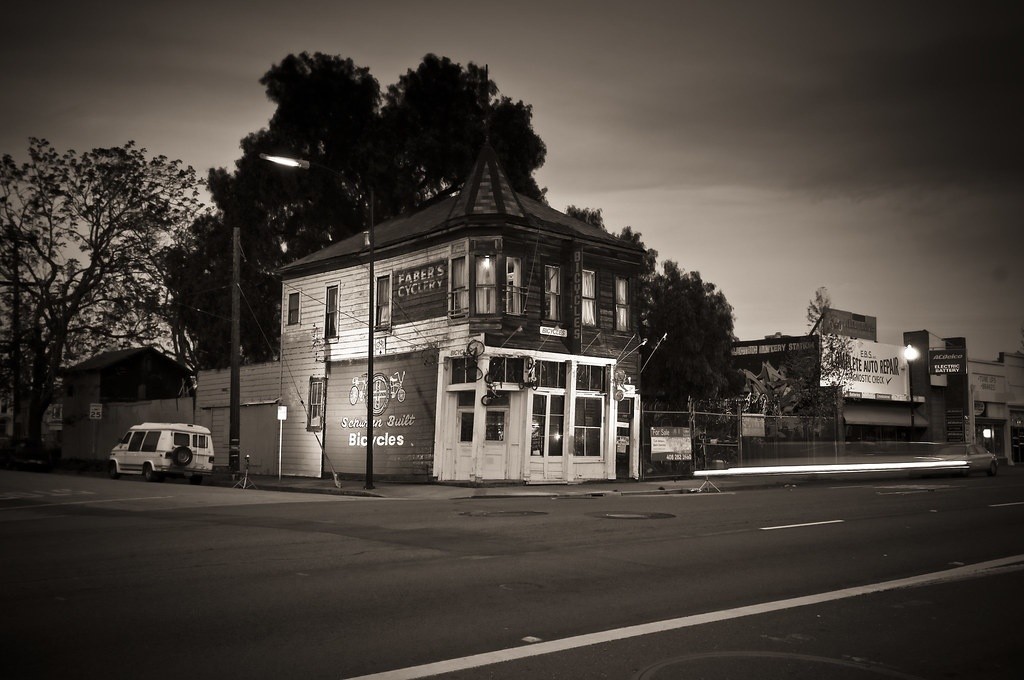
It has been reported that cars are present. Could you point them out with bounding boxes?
[931,443,998,477]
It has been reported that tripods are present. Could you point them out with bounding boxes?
[697,438,721,493]
[232,457,259,491]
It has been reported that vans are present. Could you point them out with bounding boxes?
[108,422,215,485]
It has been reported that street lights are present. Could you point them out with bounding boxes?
[259,152,377,490]
[904,343,918,441]
